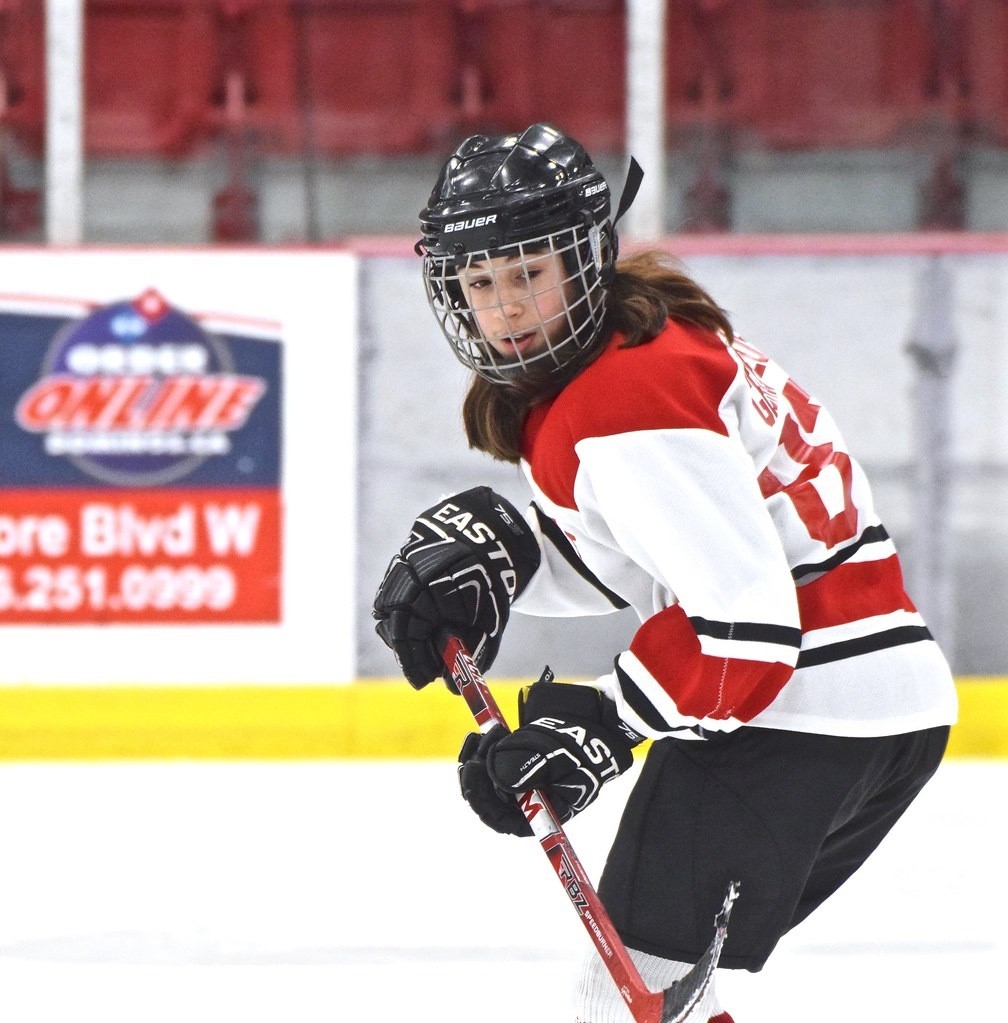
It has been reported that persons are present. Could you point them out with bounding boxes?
[371,121,958,1023]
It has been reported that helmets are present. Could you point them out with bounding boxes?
[413,121,644,389]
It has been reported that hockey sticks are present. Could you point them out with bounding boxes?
[439,636,742,1023]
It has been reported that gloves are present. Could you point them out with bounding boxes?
[374,484,541,689]
[457,664,634,834]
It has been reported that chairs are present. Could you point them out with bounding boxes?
[0,0,1008,241]
